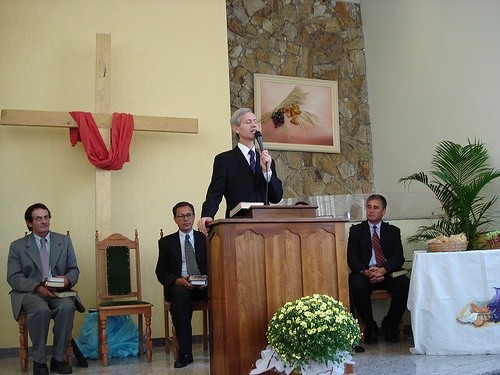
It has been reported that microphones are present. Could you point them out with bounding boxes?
[255,131,265,153]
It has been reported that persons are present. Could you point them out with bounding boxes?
[7,203,85,375]
[198,107,284,236]
[346,193,411,346]
[155,201,208,368]
[296,202,367,353]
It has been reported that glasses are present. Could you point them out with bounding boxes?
[175,213,194,221]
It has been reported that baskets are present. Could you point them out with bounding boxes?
[487,236,500,249]
[427,241,468,252]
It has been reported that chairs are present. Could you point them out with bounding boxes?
[352,222,405,343]
[18,231,73,374]
[95,229,154,366]
[160,229,208,354]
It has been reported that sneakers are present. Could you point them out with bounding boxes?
[50,358,73,374]
[33,360,49,375]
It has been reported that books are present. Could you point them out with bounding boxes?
[44,278,77,297]
[101,294,138,303]
[390,269,409,277]
[187,275,207,286]
[230,202,265,218]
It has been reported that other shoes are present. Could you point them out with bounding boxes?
[381,316,399,342]
[363,316,378,344]
[174,355,193,368]
[353,346,365,352]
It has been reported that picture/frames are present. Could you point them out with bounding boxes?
[253,73,341,155]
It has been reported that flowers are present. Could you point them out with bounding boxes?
[265,293,364,371]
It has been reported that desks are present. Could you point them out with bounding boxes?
[406,250,500,356]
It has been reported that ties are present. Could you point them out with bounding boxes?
[185,235,201,275]
[372,225,385,267]
[248,150,257,174]
[40,237,49,282]
